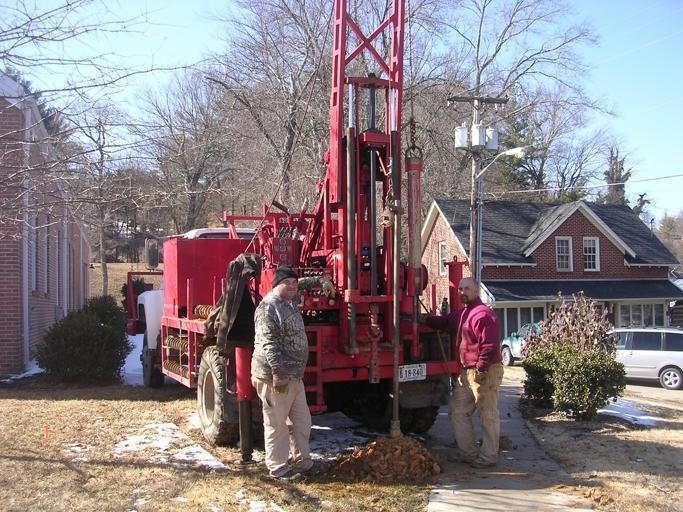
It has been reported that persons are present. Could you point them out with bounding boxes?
[414,275,506,471]
[248,263,338,482]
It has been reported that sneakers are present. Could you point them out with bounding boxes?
[447,450,480,463]
[470,457,496,469]
[296,457,331,476]
[270,469,302,481]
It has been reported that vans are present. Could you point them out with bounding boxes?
[594,329,683,390]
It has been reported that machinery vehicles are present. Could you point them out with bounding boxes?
[127,2,471,446]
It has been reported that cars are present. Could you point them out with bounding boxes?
[500,323,542,366]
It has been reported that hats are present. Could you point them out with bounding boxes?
[270,266,299,289]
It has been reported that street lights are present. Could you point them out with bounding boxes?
[446,97,512,281]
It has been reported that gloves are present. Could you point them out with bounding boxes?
[472,371,487,385]
